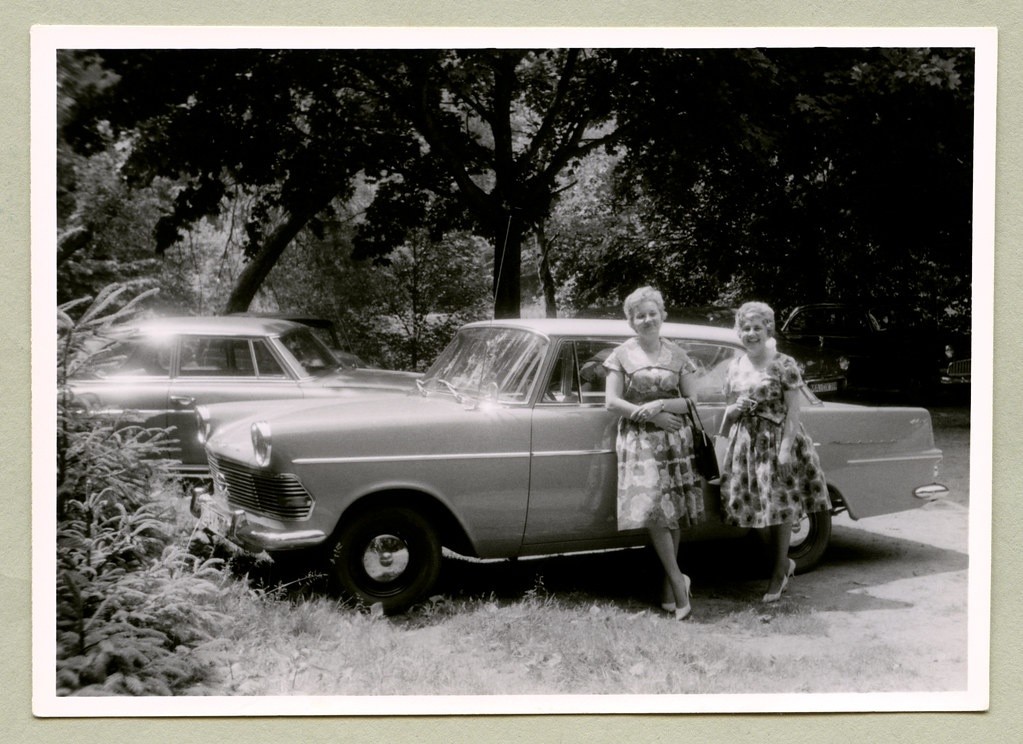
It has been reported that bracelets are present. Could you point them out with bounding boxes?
[660,400,665,409]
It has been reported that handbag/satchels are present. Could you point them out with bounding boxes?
[685,396,720,481]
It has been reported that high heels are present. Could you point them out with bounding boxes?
[786,558,795,582]
[674,575,694,622]
[760,574,789,603]
[661,602,676,614]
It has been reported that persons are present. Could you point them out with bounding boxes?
[720,301,832,602]
[602,287,706,619]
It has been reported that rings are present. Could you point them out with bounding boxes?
[643,414,646,417]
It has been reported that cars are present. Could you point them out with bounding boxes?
[56,316,432,495]
[192,315,951,623]
